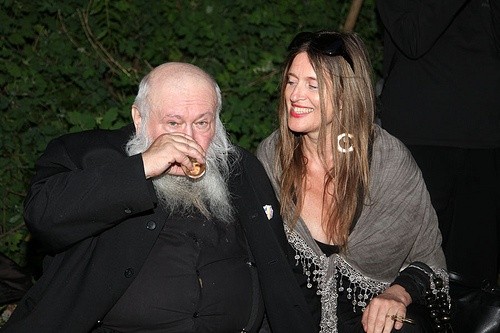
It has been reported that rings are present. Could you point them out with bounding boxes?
[386,314,415,325]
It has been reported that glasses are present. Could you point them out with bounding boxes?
[287,31,354,74]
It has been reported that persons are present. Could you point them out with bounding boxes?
[2,60,317,333]
[252,27,454,333]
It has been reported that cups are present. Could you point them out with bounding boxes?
[180,156,208,179]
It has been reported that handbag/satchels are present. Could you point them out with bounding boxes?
[402,261,500,333]
